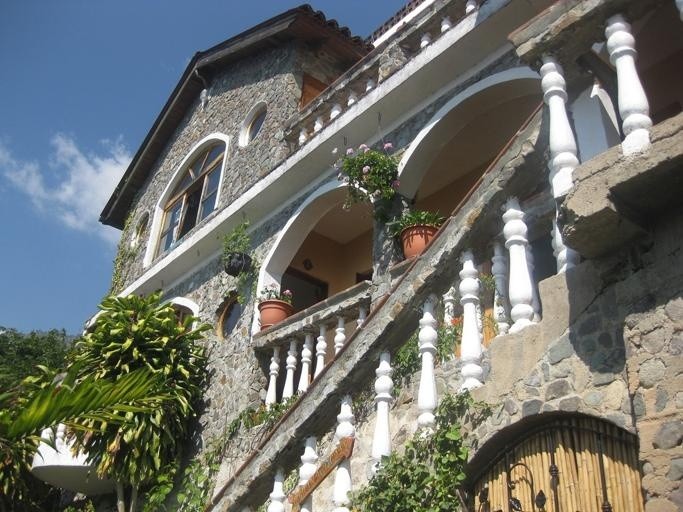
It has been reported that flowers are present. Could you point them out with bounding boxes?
[255,282,294,304]
[329,139,407,211]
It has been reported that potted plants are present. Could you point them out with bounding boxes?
[214,213,258,303]
[383,211,449,258]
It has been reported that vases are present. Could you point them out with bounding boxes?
[371,201,391,219]
[258,300,293,330]
